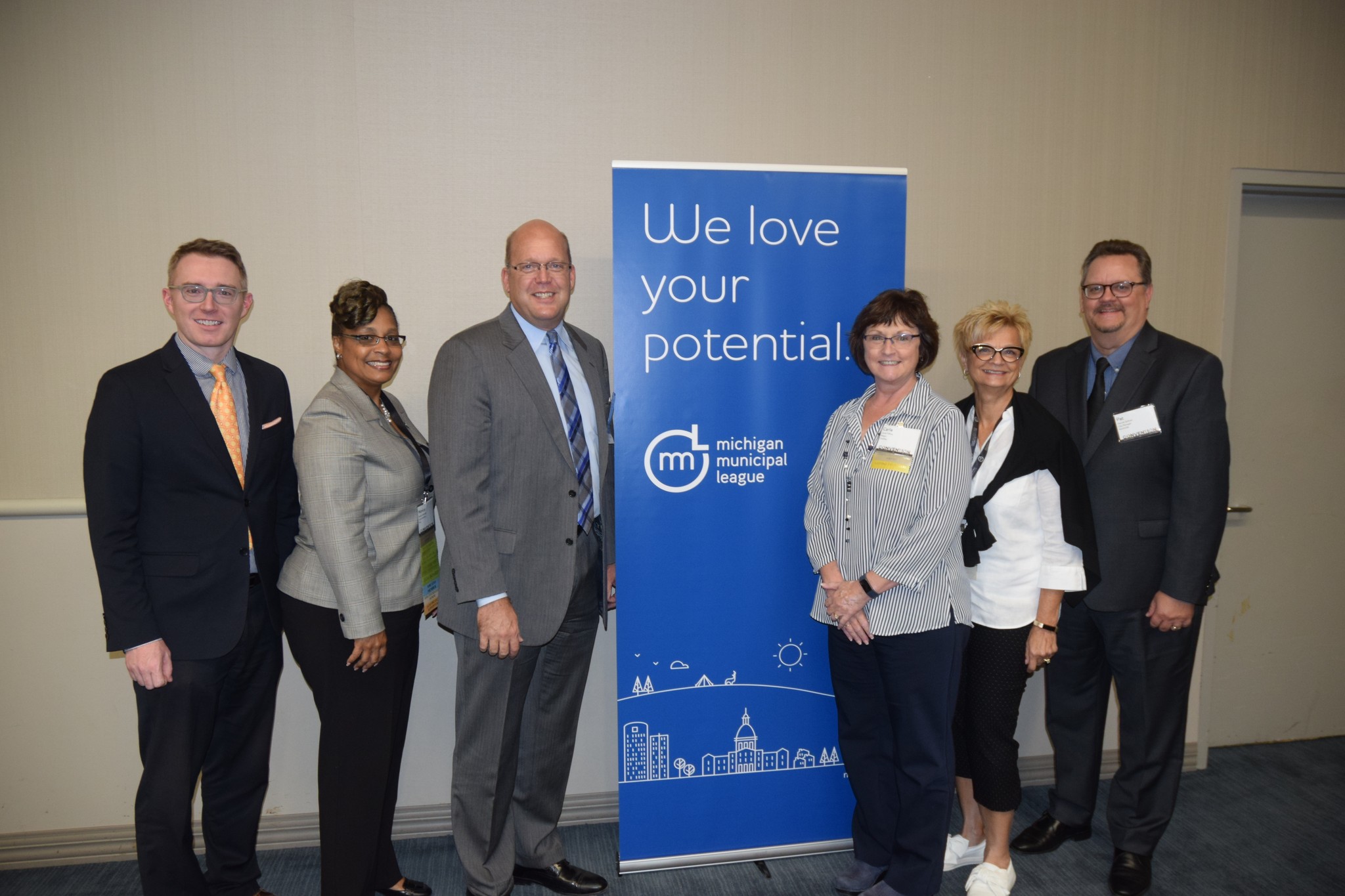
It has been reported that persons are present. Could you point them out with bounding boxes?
[1010,239,1231,896]
[804,287,972,896]
[83,238,300,896]
[943,299,1086,896]
[275,280,435,895]
[427,220,616,896]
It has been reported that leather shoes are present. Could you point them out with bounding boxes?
[513,858,608,896]
[1009,812,1092,854]
[1108,845,1153,896]
[375,875,432,896]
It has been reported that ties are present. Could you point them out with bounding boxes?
[546,330,595,536]
[1087,357,1110,440]
[210,364,245,492]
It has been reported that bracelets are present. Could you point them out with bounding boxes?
[859,573,879,599]
[1033,620,1058,633]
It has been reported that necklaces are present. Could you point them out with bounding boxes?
[379,397,393,424]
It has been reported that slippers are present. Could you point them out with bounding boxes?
[834,860,889,893]
[858,880,904,896]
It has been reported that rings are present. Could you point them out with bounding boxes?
[1043,658,1050,664]
[845,623,851,627]
[833,612,839,620]
[1172,626,1181,630]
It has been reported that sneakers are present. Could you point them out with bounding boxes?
[965,854,1017,896]
[943,833,986,872]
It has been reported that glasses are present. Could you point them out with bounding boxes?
[1081,281,1144,300]
[169,284,248,305]
[860,333,921,349]
[336,332,406,346]
[969,343,1025,363]
[506,261,570,276]
